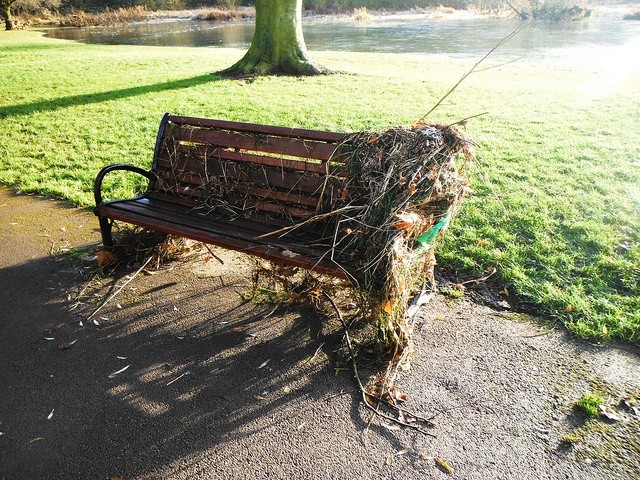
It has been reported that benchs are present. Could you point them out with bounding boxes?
[93,113,444,348]
